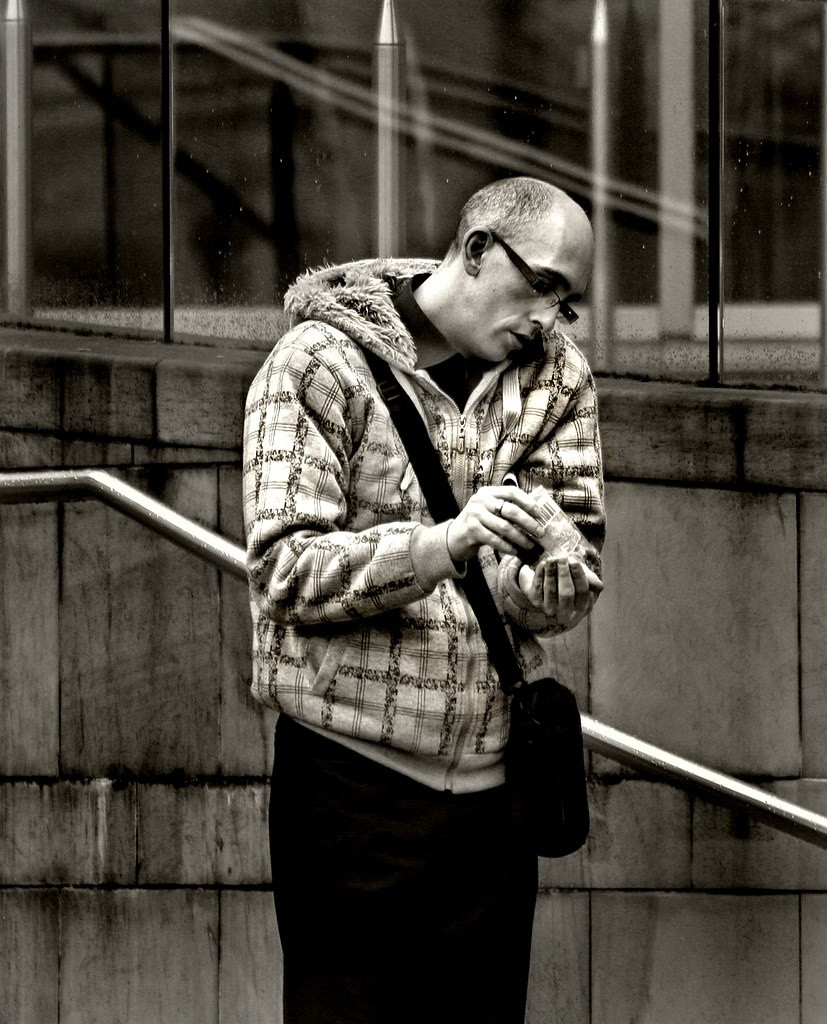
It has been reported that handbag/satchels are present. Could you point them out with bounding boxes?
[503,675,590,857]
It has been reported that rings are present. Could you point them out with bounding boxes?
[494,499,504,516]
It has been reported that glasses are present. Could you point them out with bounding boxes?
[491,231,580,326]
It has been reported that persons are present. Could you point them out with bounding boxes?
[241,178,609,1024]
